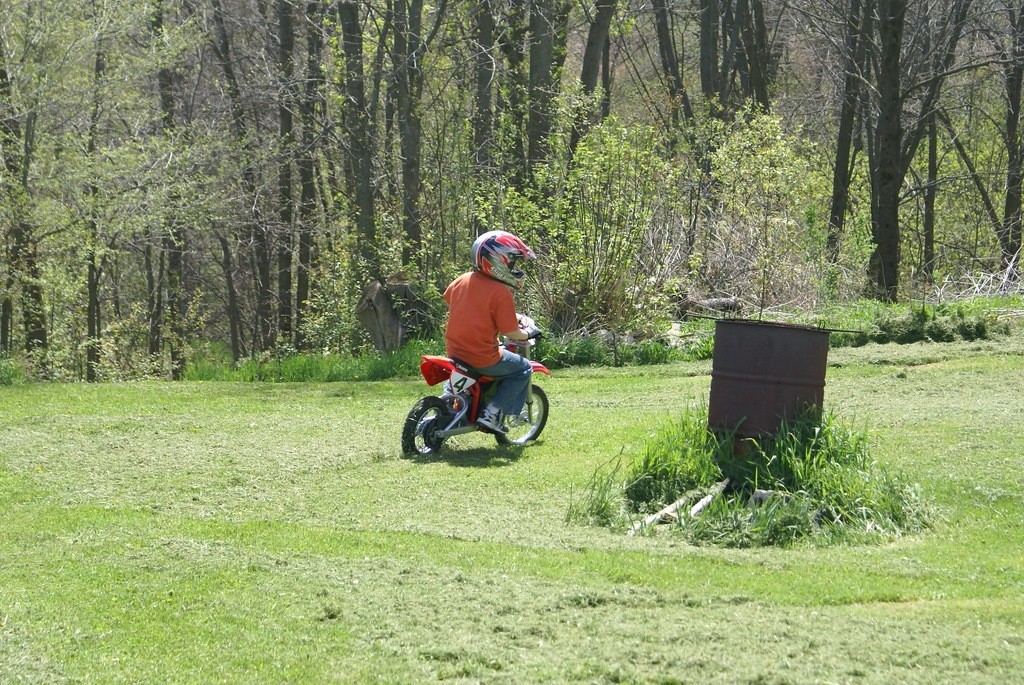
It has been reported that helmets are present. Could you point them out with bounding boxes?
[470,231,537,291]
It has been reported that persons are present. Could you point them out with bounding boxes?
[442,230,539,434]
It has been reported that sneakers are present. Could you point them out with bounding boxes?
[475,408,510,434]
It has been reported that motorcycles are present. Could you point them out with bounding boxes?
[401,312,552,456]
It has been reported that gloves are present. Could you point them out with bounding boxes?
[524,325,541,340]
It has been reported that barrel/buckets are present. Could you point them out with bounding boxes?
[707,317,832,493]
